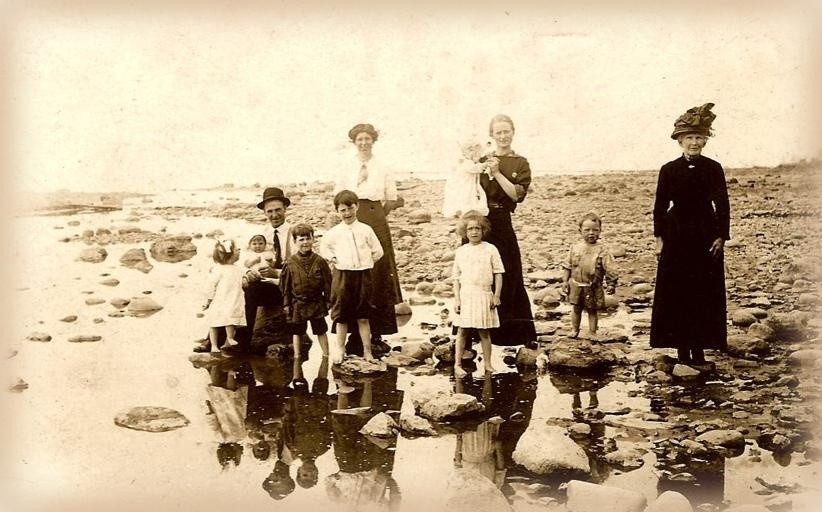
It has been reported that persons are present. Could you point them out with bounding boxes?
[447,210,506,375]
[478,113,540,349]
[206,364,406,511]
[244,234,276,286]
[441,140,492,217]
[648,132,732,366]
[657,453,727,512]
[559,212,619,338]
[331,123,405,355]
[455,415,508,490]
[233,186,300,352]
[277,223,334,358]
[320,189,384,367]
[567,389,613,490]
[196,236,249,353]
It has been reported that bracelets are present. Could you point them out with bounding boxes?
[494,170,501,179]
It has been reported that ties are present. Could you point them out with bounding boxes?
[274,230,282,266]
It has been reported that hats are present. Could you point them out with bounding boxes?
[256,186,290,210]
[671,103,716,139]
